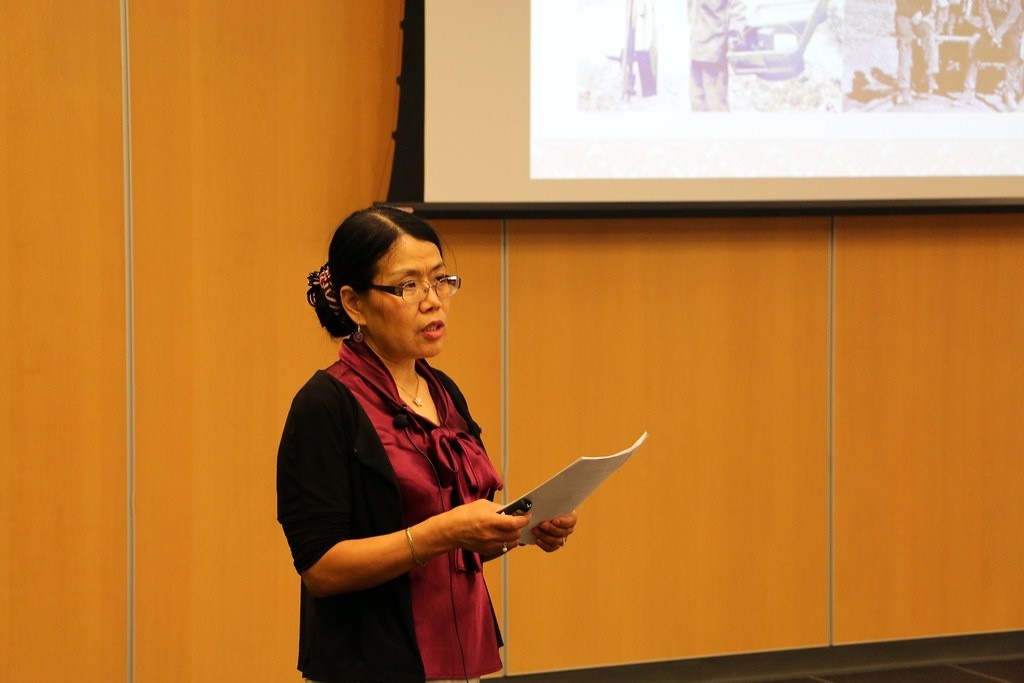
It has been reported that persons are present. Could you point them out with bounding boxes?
[613,0,660,100]
[686,0,745,114]
[276,206,578,683]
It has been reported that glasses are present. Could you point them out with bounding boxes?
[367,274,462,304]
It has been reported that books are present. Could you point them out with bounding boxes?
[494,429,648,545]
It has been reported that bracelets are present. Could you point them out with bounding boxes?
[406,527,428,568]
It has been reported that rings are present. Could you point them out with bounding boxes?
[558,537,566,548]
[502,542,508,553]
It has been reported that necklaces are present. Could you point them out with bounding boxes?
[396,373,423,407]
